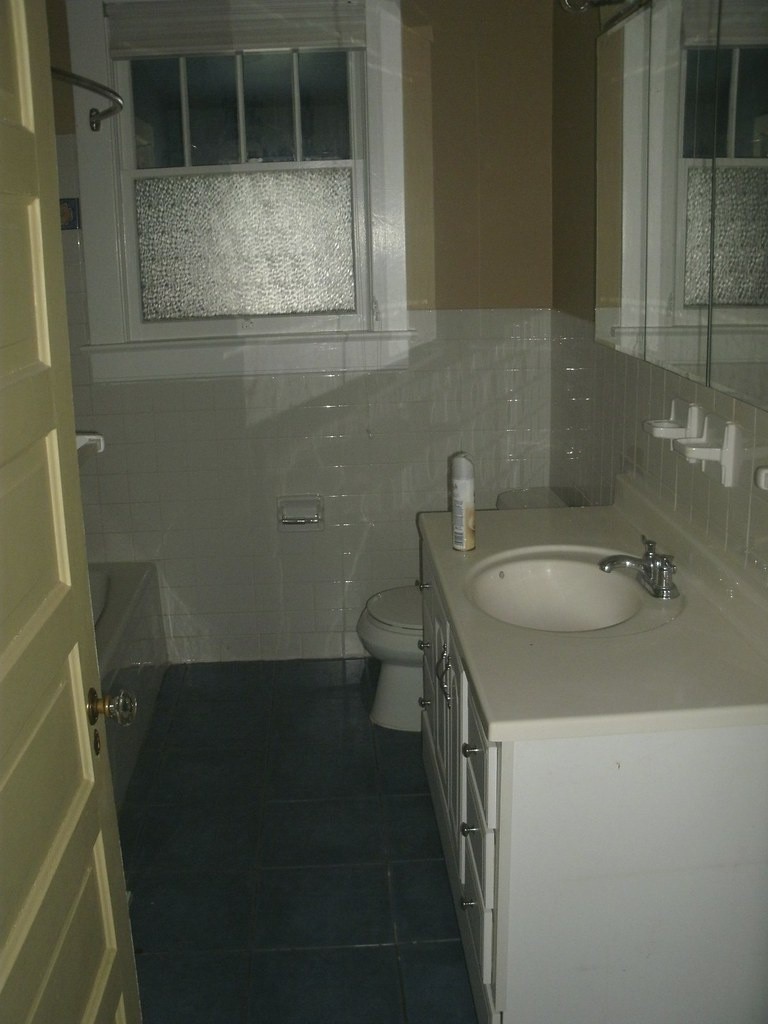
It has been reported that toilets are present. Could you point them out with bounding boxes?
[356,487,575,734]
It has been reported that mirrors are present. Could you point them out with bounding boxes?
[590,0,768,414]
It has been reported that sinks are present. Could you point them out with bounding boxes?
[466,557,650,633]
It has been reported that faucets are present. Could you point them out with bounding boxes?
[599,535,681,601]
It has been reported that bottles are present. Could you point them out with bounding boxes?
[450,452,476,551]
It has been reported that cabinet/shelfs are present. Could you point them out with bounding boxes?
[420,537,768,1024]
